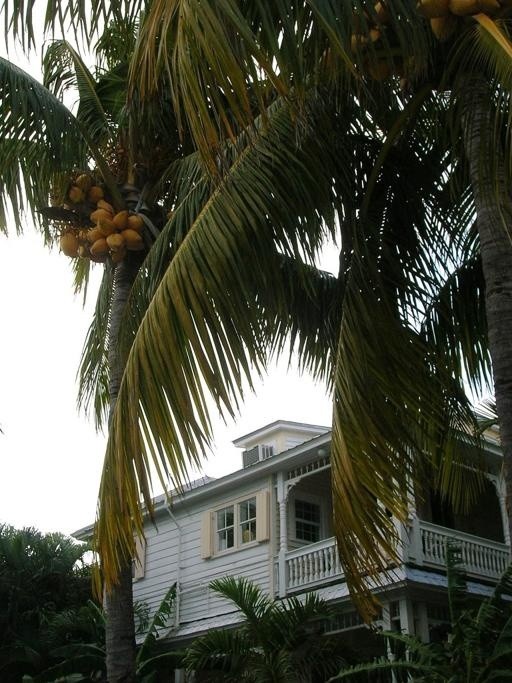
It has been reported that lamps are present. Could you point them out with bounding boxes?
[316,448,326,457]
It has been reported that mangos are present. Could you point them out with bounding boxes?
[58,173,145,263]
